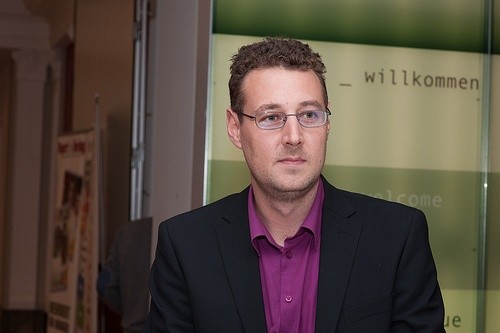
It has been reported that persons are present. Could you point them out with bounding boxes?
[150,38,447,333]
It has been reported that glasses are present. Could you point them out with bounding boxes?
[233,107,332,129]
